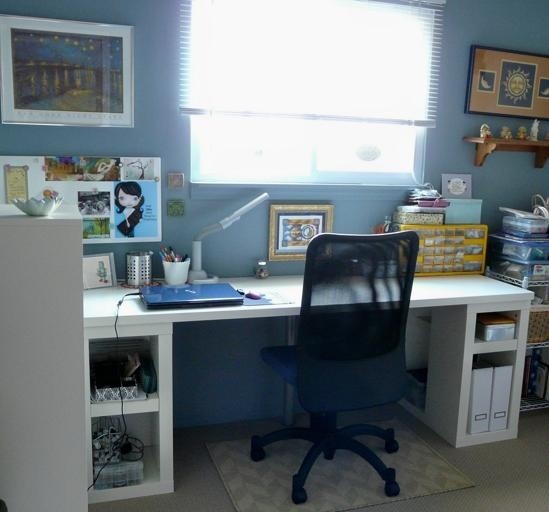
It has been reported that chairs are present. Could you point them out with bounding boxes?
[253,233,420,505]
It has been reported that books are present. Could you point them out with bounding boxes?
[523,348,548,399]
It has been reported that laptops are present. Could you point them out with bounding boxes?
[139,283,244,309]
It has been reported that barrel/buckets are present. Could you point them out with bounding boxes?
[12,60,123,108]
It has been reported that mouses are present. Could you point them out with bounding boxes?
[245,290,262,299]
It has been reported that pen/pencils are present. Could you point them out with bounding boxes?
[159,246,188,261]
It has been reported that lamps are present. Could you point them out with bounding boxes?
[187,193,270,282]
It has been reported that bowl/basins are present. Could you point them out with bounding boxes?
[12,196,65,216]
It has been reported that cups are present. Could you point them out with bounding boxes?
[162,257,190,287]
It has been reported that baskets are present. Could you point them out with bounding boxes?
[503,309,549,345]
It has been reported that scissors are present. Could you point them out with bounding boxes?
[377,223,400,234]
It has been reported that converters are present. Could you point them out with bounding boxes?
[120,440,132,455]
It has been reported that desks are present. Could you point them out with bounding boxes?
[487,267,549,411]
[0,203,90,512]
[83,273,536,505]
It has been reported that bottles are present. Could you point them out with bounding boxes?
[384,215,392,232]
[255,261,271,278]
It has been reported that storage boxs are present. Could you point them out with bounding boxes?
[487,250,549,284]
[503,217,549,237]
[483,323,515,341]
[392,222,488,276]
[442,199,482,224]
[488,234,549,262]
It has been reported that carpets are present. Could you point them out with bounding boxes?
[208,422,475,510]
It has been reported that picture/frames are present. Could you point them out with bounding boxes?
[269,203,334,261]
[465,43,549,121]
[0,14,134,128]
[83,252,117,289]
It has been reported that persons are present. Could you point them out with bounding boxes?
[96,261,108,283]
[78,193,110,218]
